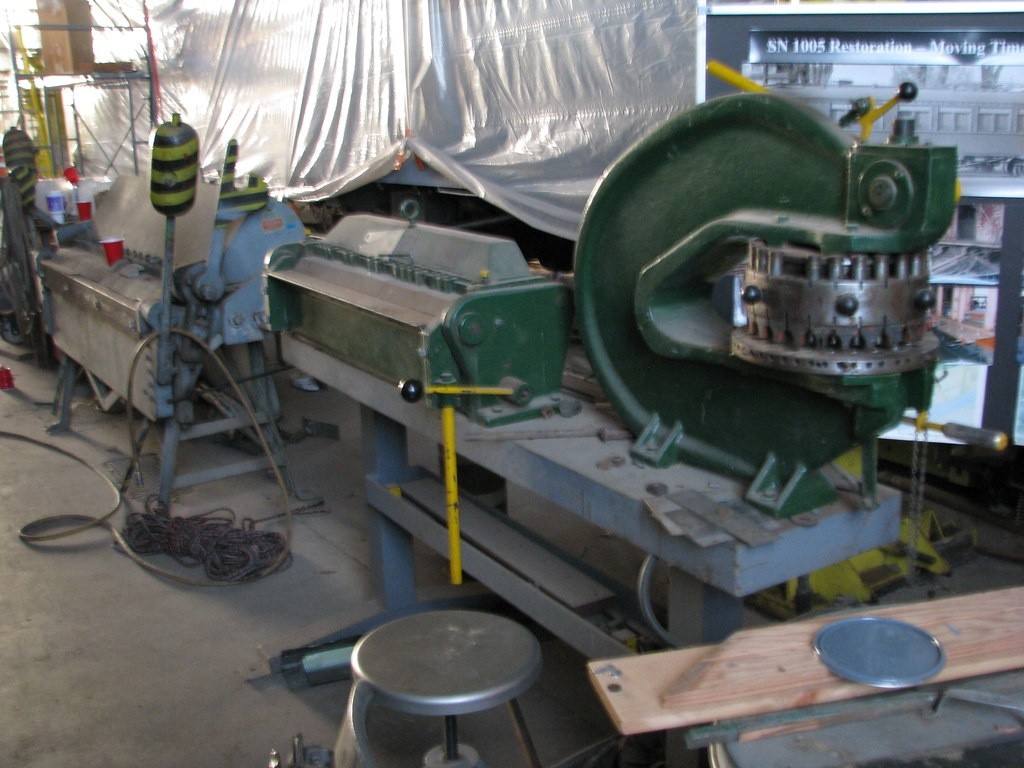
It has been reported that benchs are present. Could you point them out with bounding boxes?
[587,583,1023,768]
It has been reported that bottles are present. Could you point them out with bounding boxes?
[34,178,97,225]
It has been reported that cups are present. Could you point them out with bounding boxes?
[76,201,92,221]
[100,237,124,268]
[64,167,79,184]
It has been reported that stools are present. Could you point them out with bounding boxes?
[346,610,544,768]
